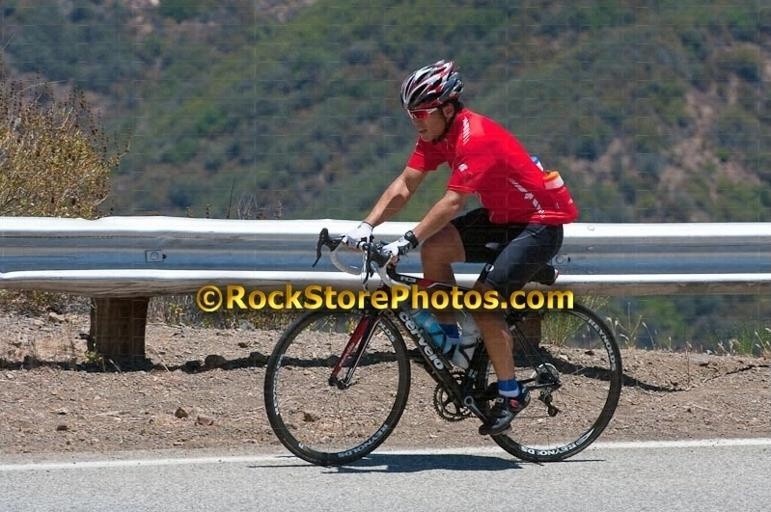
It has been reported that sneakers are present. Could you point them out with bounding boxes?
[409,345,459,364]
[476,384,535,437]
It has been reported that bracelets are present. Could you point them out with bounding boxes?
[404,230,419,249]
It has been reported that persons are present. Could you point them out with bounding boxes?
[346,57,581,434]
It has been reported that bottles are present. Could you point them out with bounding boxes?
[531,156,544,172]
[412,306,453,356]
[450,314,484,370]
[545,169,579,222]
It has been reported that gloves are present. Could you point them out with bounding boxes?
[342,221,376,250]
[380,229,421,260]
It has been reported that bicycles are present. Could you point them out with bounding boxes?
[264,229,623,466]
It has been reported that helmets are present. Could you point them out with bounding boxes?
[398,60,464,114]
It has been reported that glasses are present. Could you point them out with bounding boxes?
[407,107,442,122]
